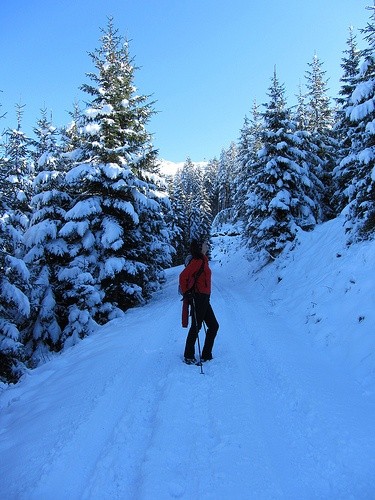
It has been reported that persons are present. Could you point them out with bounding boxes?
[179,238,220,365]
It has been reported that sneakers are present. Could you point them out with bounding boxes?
[183,358,203,366]
[201,356,214,362]
